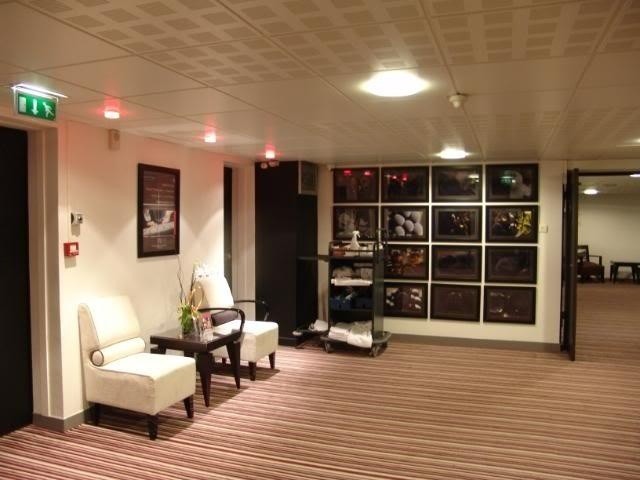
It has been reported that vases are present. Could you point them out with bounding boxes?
[178,303,196,334]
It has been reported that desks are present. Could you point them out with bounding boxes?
[609,259,640,284]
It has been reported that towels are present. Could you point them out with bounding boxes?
[309,318,373,349]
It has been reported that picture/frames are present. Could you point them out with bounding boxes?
[133,162,182,259]
[330,161,541,327]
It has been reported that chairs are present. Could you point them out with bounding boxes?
[78,294,198,441]
[577,244,605,284]
[191,274,281,381]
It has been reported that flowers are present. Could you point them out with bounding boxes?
[173,253,211,331]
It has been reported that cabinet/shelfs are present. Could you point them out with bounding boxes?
[294,240,391,357]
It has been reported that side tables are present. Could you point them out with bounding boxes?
[151,325,242,408]
